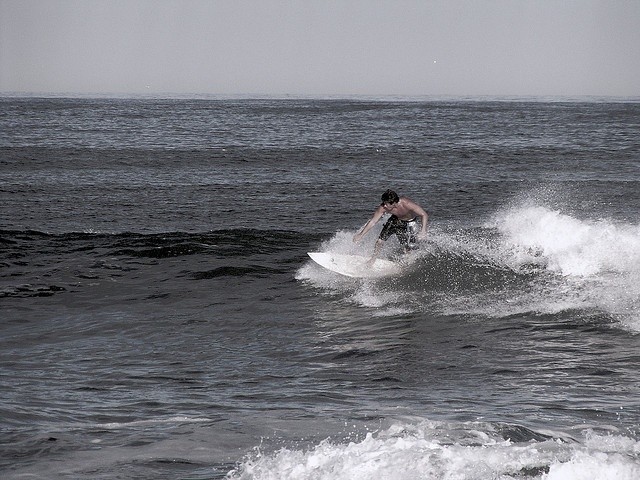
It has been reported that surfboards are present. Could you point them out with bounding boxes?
[307,252,400,278]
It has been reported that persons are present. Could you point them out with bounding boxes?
[352,188,429,268]
